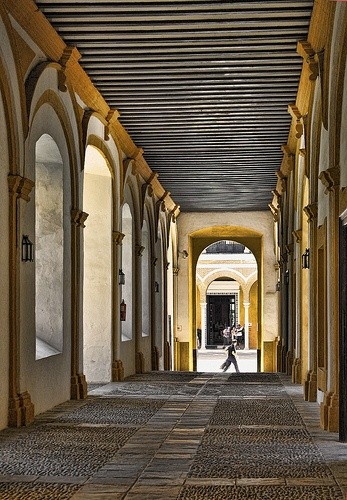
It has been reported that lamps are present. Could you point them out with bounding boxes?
[22,235,35,263]
[118,269,125,285]
[301,248,310,269]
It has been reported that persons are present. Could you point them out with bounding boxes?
[222,339,239,372]
[221,323,245,351]
